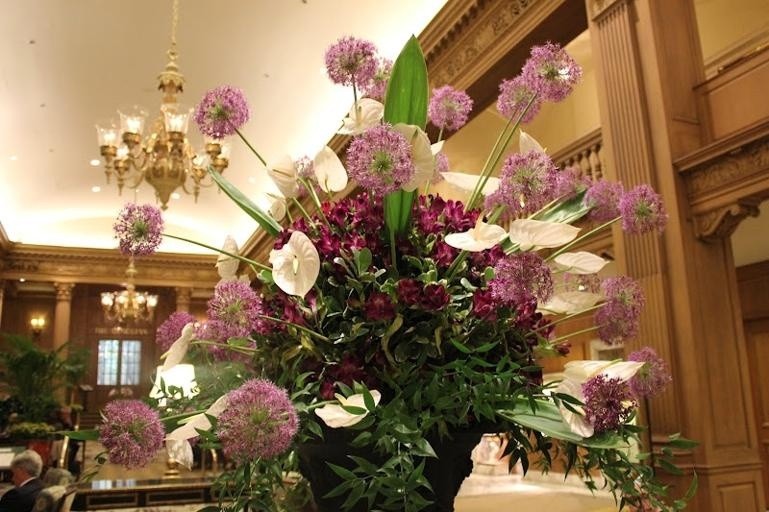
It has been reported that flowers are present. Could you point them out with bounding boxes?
[99,32,701,512]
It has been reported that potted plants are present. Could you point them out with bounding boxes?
[3,334,92,467]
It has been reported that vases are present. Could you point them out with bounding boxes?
[294,417,485,511]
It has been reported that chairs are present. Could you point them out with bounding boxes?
[31,469,79,511]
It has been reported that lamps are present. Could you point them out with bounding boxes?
[101,249,159,335]
[97,5,231,212]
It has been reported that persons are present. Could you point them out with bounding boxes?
[0,449,50,512]
